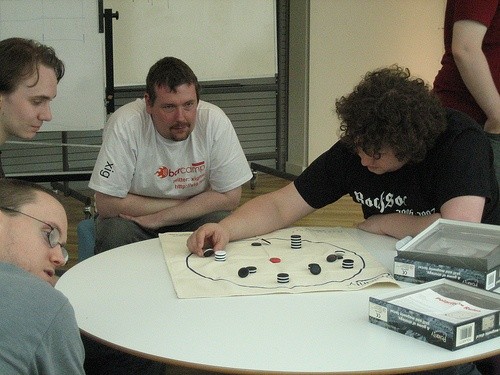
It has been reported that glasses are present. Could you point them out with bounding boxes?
[0,207,68,266]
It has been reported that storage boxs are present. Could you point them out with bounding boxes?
[368,218,500,351]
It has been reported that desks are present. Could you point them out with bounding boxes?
[53,227,500,375]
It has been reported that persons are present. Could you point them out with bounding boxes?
[0,37,65,178]
[429,0,500,133]
[0,179,85,375]
[186,67,500,257]
[88,57,253,255]
